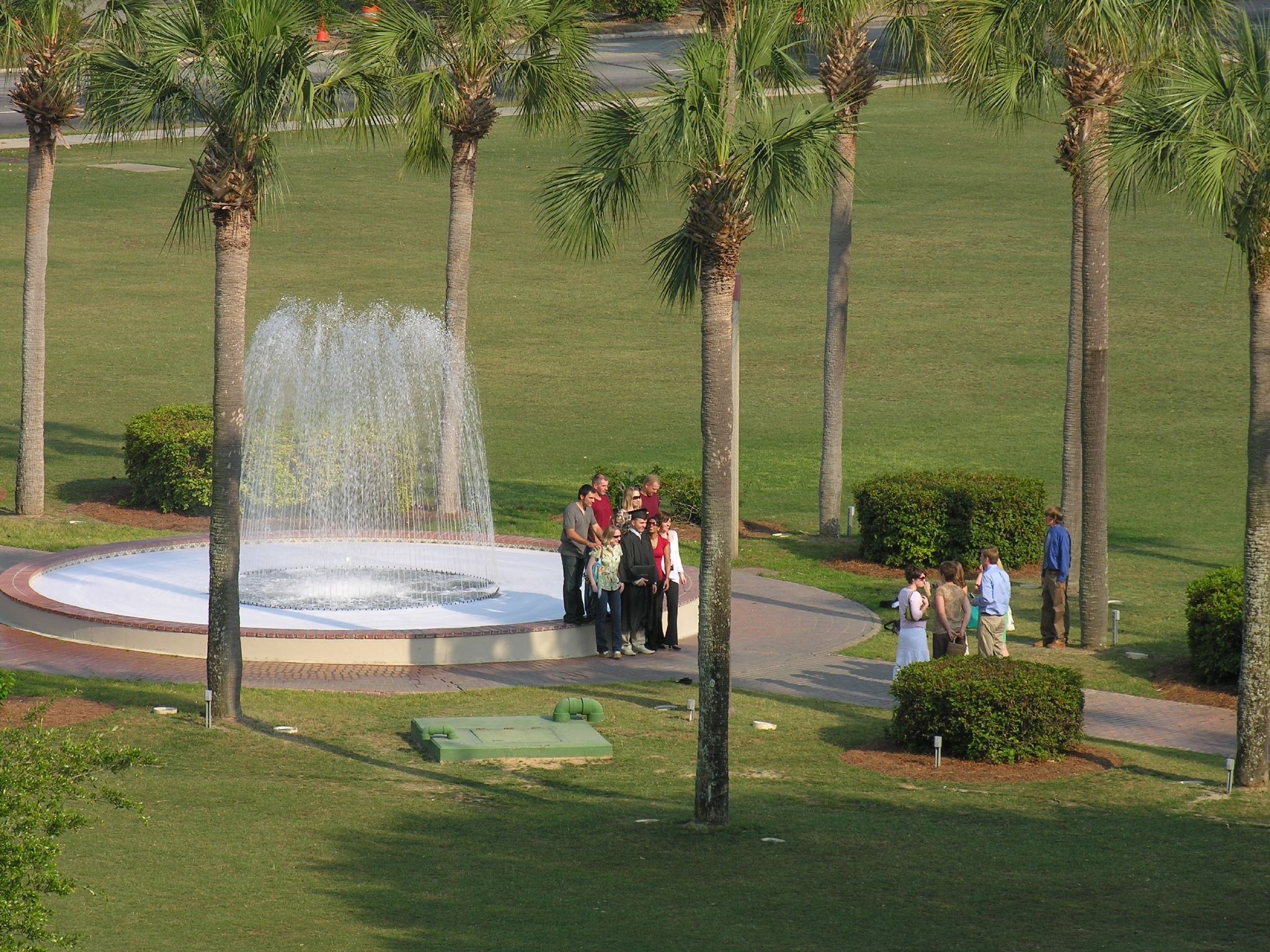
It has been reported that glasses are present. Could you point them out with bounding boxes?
[614,535,621,538]
[648,521,658,526]
[633,496,641,499]
[919,576,925,581]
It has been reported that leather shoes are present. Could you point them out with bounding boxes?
[622,649,636,656]
[637,647,655,654]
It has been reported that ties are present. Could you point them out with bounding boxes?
[1044,531,1052,568]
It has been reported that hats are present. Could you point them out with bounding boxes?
[627,508,650,520]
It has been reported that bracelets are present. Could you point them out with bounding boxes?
[679,574,684,577]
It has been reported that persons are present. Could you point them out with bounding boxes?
[923,548,1011,659]
[893,567,930,681]
[558,484,604,625]
[1032,506,1070,648]
[584,474,687,658]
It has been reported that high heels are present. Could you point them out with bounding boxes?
[669,644,681,649]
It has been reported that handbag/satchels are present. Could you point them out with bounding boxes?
[946,633,967,656]
[583,546,603,582]
[906,591,931,621]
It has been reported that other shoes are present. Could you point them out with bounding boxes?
[599,651,613,658]
[574,617,592,624]
[1047,642,1065,649]
[1033,640,1048,646]
[613,652,622,658]
